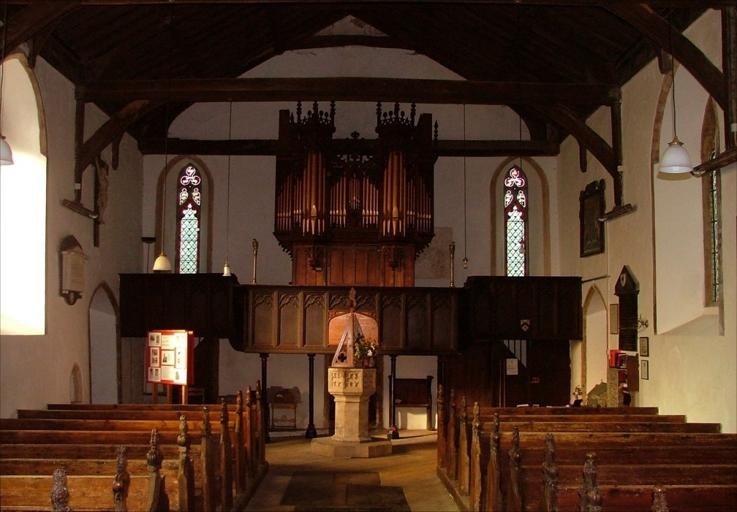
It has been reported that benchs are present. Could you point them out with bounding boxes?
[0,381,265,511]
[434,386,736,511]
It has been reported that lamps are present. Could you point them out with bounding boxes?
[222,101,233,278]
[462,103,468,269]
[151,129,173,271]
[658,52,693,175]
[1,48,14,167]
[518,112,525,253]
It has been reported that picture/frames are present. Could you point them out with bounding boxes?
[640,360,649,380]
[638,336,649,357]
[578,179,605,258]
[608,303,619,334]
